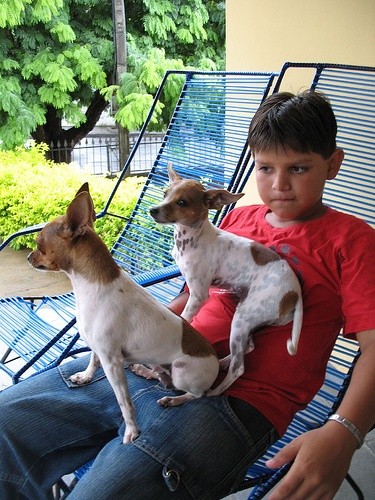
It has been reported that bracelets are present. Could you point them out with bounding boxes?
[328,413,364,448]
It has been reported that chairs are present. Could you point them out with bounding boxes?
[0,61,375,500]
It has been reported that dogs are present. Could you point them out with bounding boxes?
[27,182,219,442]
[149,163,304,397]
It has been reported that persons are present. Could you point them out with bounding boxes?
[0,90,375,500]
